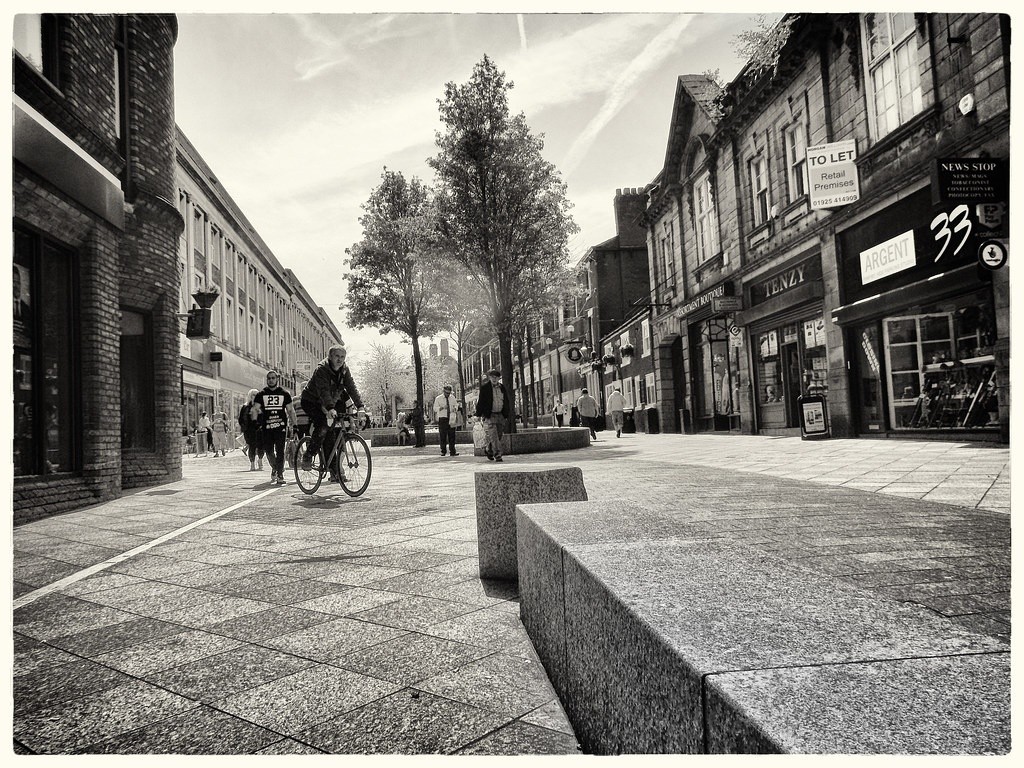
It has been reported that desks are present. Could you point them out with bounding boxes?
[193,431,209,457]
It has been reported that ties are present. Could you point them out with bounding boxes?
[446,396,450,418]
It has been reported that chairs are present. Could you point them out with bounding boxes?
[182,436,189,456]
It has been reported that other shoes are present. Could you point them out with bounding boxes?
[450,452,459,456]
[441,450,446,456]
[242,450,247,456]
[617,430,620,438]
[214,453,219,457]
[250,462,255,472]
[301,452,312,471]
[496,458,503,462]
[222,451,225,456]
[484,449,494,460]
[277,473,286,484]
[257,459,263,469]
[591,433,596,439]
[271,468,277,482]
[330,472,347,482]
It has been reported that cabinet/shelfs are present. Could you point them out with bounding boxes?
[882,302,1000,430]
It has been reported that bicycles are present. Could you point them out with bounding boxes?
[293,414,373,497]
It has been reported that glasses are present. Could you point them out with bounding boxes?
[267,377,276,380]
[444,391,451,394]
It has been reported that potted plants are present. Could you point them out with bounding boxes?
[191,285,220,309]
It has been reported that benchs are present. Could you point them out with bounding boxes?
[515,498,1012,755]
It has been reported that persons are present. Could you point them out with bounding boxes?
[371,418,377,428]
[210,405,229,457]
[553,399,566,427]
[302,346,365,483]
[396,413,409,445]
[433,386,460,456]
[578,388,598,440]
[199,411,212,451]
[606,387,627,438]
[252,371,297,484]
[410,401,424,448]
[476,371,509,461]
[238,389,265,472]
[288,381,311,449]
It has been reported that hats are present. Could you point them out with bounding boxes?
[615,387,620,391]
[489,370,501,377]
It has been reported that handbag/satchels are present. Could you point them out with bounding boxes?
[449,410,456,425]
[288,431,302,468]
[554,406,557,412]
[473,419,490,448]
[450,408,463,428]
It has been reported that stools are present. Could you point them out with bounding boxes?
[474,463,587,583]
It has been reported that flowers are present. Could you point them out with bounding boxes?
[602,354,616,367]
[592,360,603,375]
[619,344,635,358]
